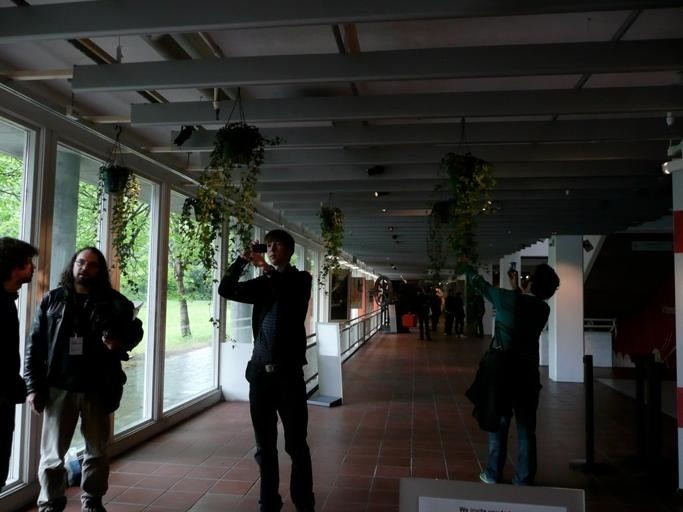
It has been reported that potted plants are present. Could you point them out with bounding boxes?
[171,85,289,304]
[88,123,154,299]
[316,192,351,294]
[422,120,503,278]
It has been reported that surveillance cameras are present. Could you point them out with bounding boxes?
[174,129,194,146]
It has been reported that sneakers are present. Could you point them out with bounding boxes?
[511,475,528,486]
[479,471,497,484]
[417,326,465,341]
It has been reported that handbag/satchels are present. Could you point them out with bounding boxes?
[402,312,416,327]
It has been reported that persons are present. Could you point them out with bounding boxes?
[454,255,559,486]
[407,286,484,343]
[22,245,143,512]
[213,227,317,511]
[0,237,42,492]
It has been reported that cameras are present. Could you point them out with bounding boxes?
[253,244,267,253]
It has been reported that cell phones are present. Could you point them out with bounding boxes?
[509,262,516,273]
